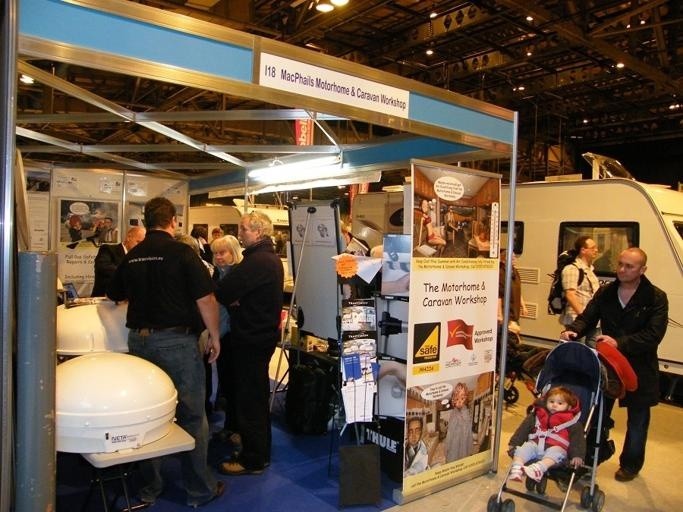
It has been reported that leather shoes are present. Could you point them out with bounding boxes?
[615,467,636,481]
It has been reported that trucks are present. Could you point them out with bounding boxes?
[186,202,294,293]
[351,155,682,402]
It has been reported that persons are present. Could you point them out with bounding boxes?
[495,250,526,384]
[90,197,383,511]
[67,215,82,242]
[508,387,586,484]
[559,246,668,481]
[557,236,616,427]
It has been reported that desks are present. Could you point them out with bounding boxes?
[84,424,196,512]
[269,343,356,442]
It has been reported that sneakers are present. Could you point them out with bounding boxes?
[521,463,544,484]
[204,397,270,496]
[510,464,522,481]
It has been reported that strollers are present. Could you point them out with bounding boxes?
[485,335,612,512]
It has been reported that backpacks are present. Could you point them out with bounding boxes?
[547,249,584,315]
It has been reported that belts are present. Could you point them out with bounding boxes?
[136,326,176,333]
